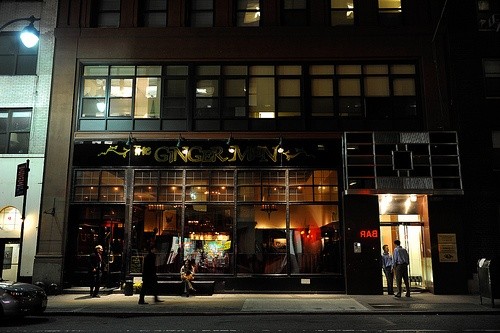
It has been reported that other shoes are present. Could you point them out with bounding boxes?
[138,301,149,304]
[191,288,197,293]
[154,298,164,302]
[90,293,100,298]
[405,294,410,297]
[395,294,401,297]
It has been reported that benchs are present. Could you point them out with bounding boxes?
[158,280,216,295]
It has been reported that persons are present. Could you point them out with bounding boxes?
[392,240,410,298]
[89,245,196,305]
[381,245,395,295]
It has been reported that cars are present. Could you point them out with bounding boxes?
[0,280,48,325]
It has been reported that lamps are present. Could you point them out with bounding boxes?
[176,133,193,154]
[125,132,142,166]
[227,128,244,154]
[277,136,285,167]
